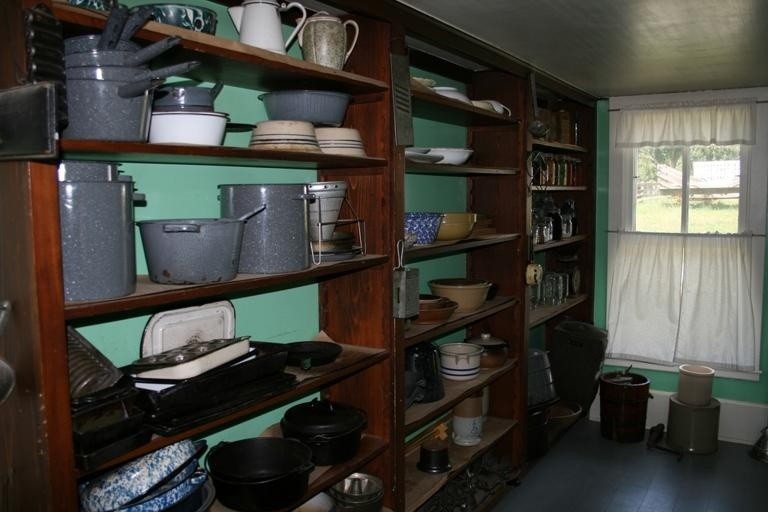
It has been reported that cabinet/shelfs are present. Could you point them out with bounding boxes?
[0,1,596,512]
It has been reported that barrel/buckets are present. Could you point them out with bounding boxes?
[217,182,315,274]
[329,473,385,512]
[58,158,145,303]
[57,162,147,303]
[527,349,560,412]
[676,364,716,405]
[599,371,654,444]
[138,218,244,285]
[304,180,345,250]
[665,395,720,456]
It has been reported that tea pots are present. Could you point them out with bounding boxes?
[227,1,359,70]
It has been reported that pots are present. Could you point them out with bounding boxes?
[61,5,202,145]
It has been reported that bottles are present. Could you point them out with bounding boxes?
[539,153,583,186]
[540,272,569,306]
[534,196,578,246]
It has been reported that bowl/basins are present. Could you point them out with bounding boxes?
[544,403,582,428]
[150,79,367,159]
[331,472,384,511]
[437,332,512,382]
[140,4,218,37]
[427,147,474,167]
[409,277,494,325]
[404,211,478,246]
[204,398,368,511]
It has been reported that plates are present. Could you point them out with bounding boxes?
[290,341,342,368]
[404,144,445,167]
